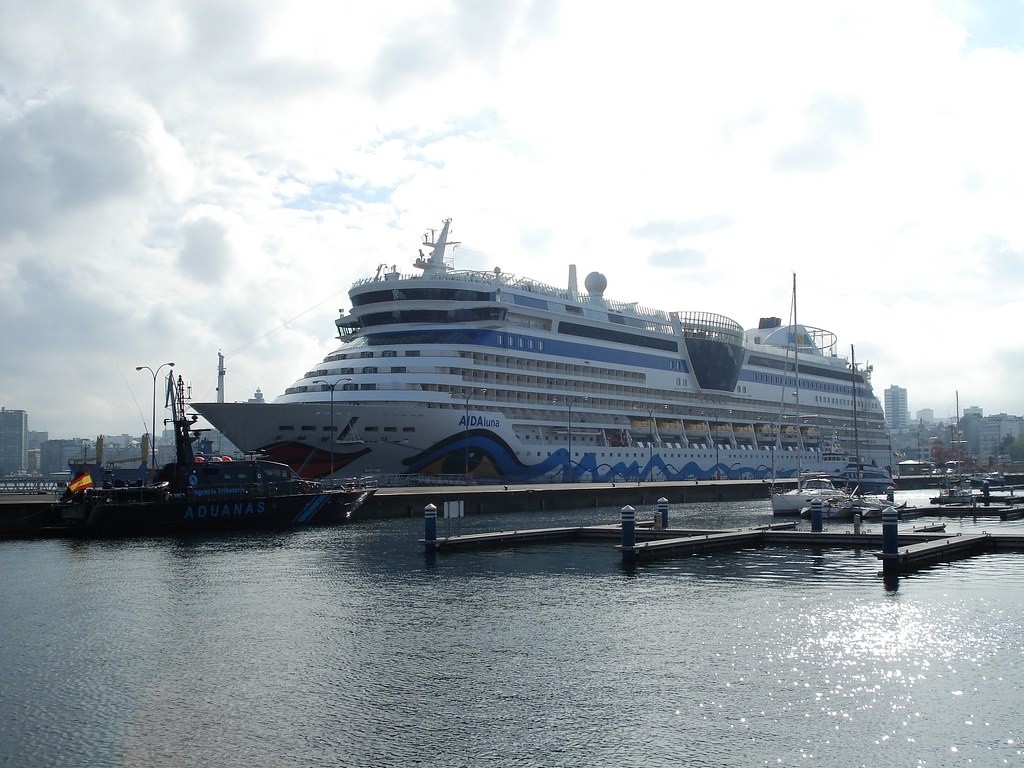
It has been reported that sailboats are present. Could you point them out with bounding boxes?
[800,345,898,496]
[940,391,1005,503]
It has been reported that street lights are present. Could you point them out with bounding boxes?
[701,410,732,480]
[803,418,831,470]
[757,416,787,482]
[632,404,668,482]
[552,396,588,483]
[842,420,868,462]
[136,363,175,483]
[312,377,354,491]
[877,424,901,470]
[1007,427,1014,474]
[448,388,486,486]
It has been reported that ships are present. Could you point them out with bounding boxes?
[187,216,893,482]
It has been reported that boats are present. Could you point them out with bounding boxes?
[631,419,656,435]
[685,423,708,437]
[757,425,779,442]
[768,475,908,520]
[801,427,820,446]
[49,375,378,536]
[734,426,755,439]
[780,425,799,443]
[710,425,732,438]
[658,422,684,436]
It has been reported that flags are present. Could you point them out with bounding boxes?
[69,472,93,492]
